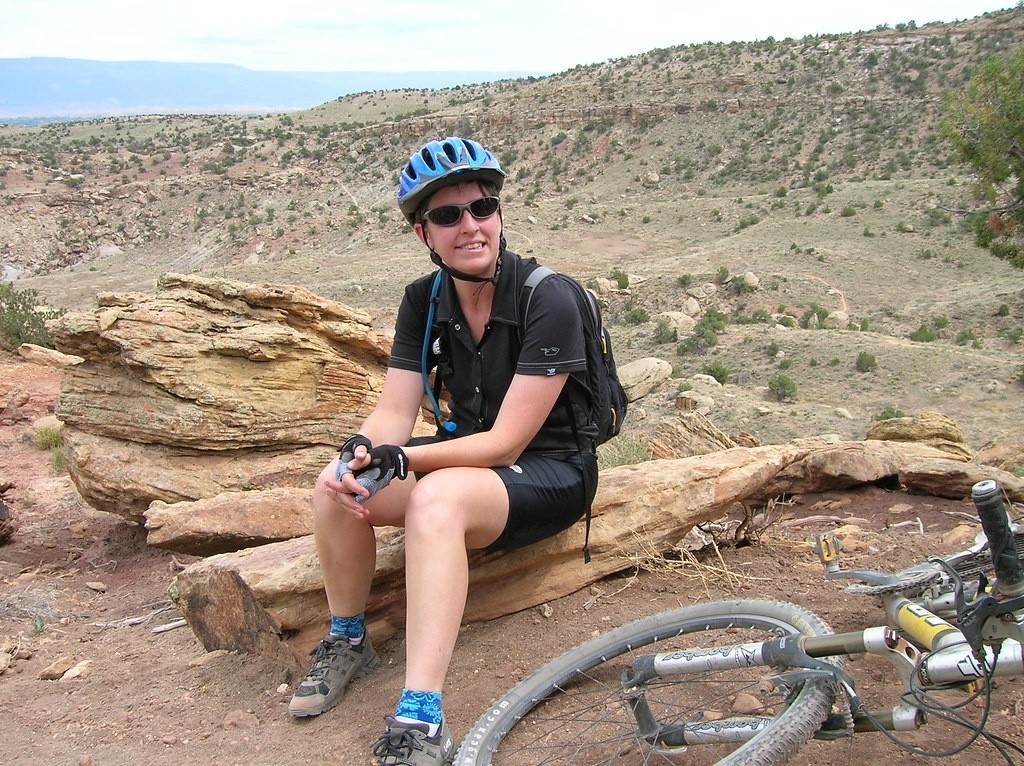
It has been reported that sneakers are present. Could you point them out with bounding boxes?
[369,714,455,766]
[289,627,381,716]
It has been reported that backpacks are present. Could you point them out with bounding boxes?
[426,266,629,448]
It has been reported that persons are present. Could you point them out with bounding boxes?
[288,137,599,766]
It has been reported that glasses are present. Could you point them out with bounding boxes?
[420,197,501,227]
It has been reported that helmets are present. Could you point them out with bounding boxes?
[397,136,506,227]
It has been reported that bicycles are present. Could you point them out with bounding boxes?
[449,480,1024,765]
